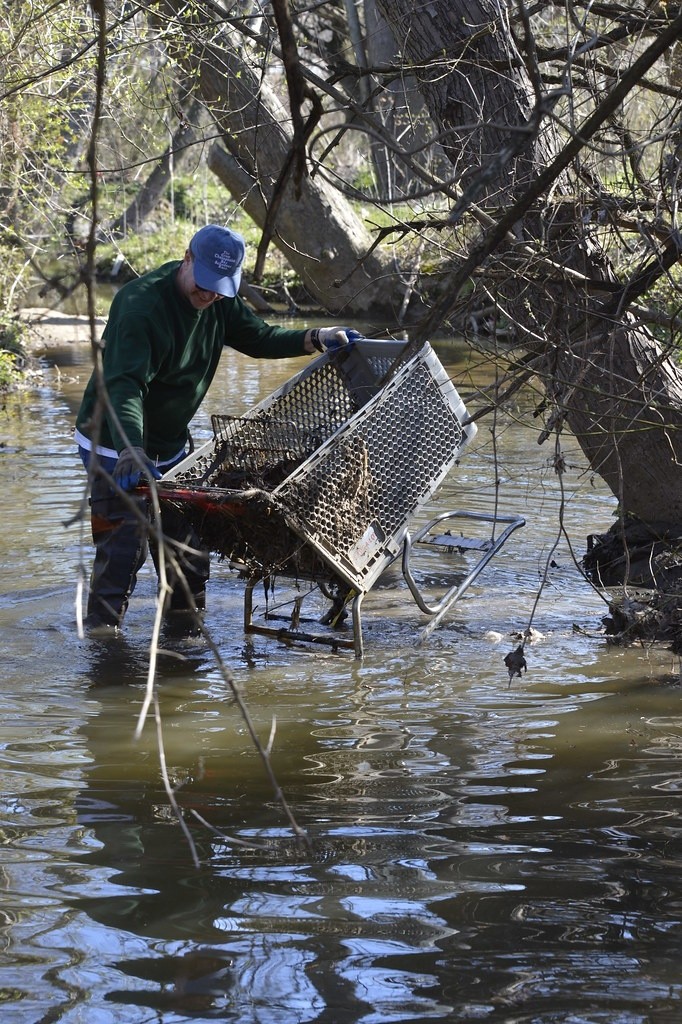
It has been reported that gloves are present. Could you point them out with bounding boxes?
[110,446,162,492]
[311,326,366,353]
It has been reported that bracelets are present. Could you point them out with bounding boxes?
[311,329,323,353]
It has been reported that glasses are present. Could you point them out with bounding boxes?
[195,283,224,296]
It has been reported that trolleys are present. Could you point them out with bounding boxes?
[137,337,527,661]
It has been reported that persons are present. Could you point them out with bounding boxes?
[73,225,362,637]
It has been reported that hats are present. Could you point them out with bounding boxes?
[190,224,244,298]
